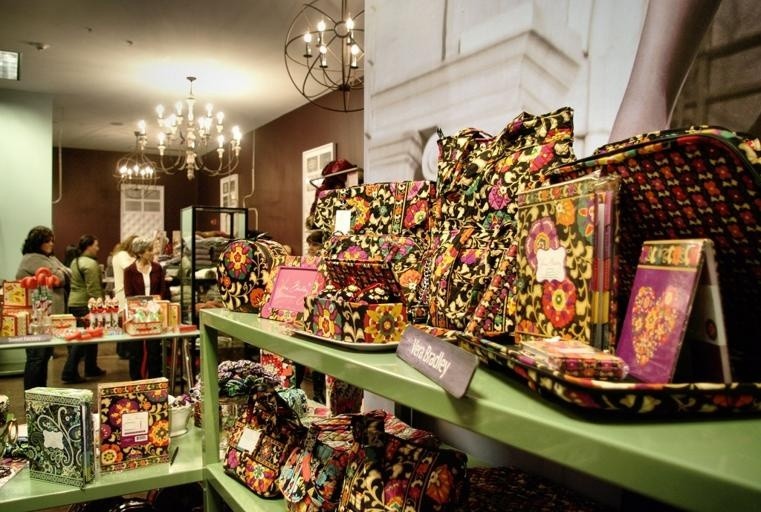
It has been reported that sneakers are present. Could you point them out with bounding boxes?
[60,370,87,384]
[84,364,107,381]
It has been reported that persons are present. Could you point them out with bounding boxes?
[107,243,123,298]
[293,230,327,405]
[123,236,168,381]
[113,234,140,357]
[58,232,106,382]
[15,225,75,388]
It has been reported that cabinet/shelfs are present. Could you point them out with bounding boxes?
[180,203,248,332]
[0,306,761,510]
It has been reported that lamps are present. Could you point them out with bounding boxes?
[285,1,365,112]
[113,75,243,199]
